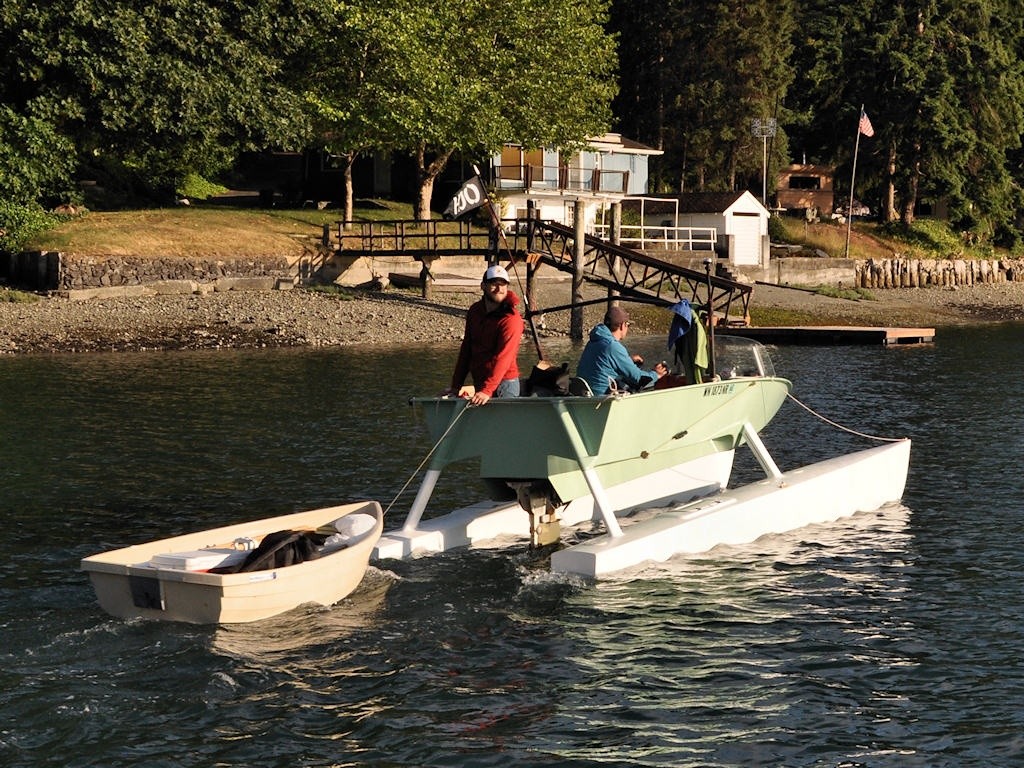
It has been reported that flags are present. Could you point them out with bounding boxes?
[441,175,488,223]
[858,108,874,137]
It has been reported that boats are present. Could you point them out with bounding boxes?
[79,500,383,625]
[369,260,912,578]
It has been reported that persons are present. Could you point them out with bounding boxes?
[444,266,523,503]
[573,307,667,397]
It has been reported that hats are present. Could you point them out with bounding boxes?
[483,265,510,284]
[605,307,635,326]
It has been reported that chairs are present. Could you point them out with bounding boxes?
[569,376,594,396]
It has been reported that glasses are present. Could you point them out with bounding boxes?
[490,282,506,288]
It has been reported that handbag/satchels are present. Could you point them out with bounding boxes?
[530,360,570,396]
